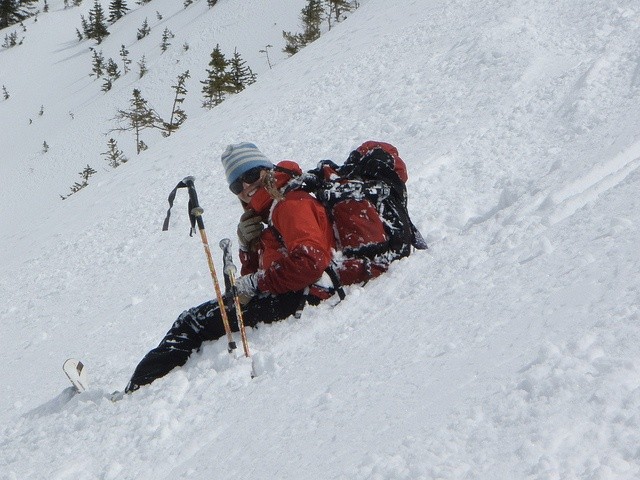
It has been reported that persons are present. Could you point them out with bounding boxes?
[113,142,338,401]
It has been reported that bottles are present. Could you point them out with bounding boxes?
[221,141,273,185]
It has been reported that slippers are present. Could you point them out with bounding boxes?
[266,141,428,319]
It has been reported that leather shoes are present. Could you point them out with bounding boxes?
[237,209,263,251]
[235,273,257,308]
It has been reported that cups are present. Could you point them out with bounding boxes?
[229,167,265,195]
